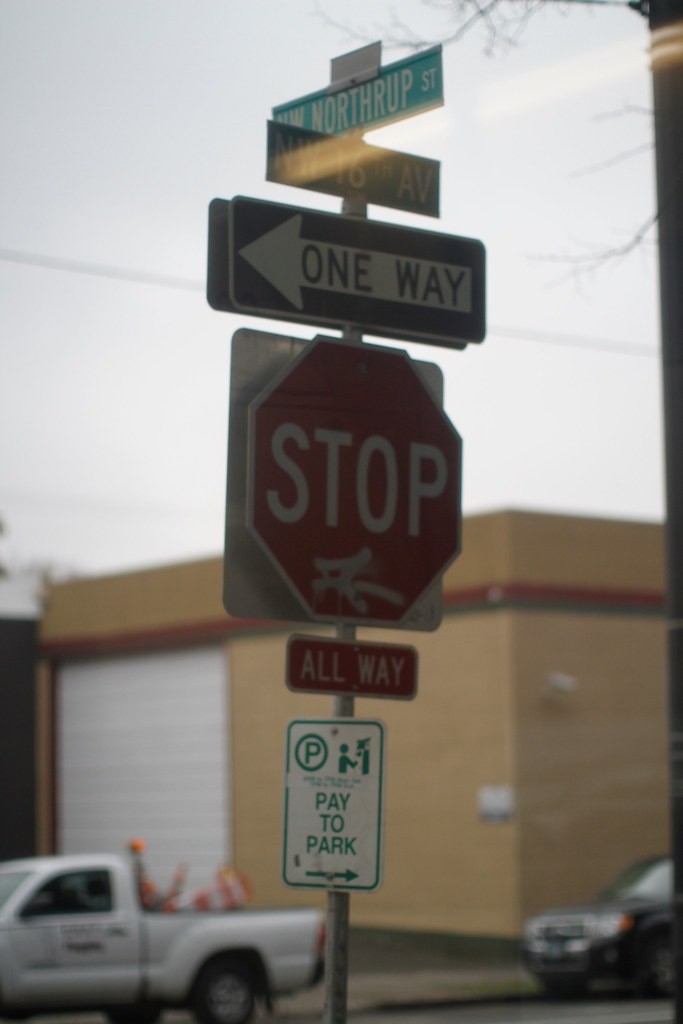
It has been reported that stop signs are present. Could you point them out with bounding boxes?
[247,334,462,624]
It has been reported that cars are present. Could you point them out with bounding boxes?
[513,851,679,1002]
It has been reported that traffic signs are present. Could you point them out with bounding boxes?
[265,120,440,218]
[282,634,420,702]
[224,193,487,347]
[273,42,443,138]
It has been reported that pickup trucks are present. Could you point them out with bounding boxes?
[0,852,325,1024]
